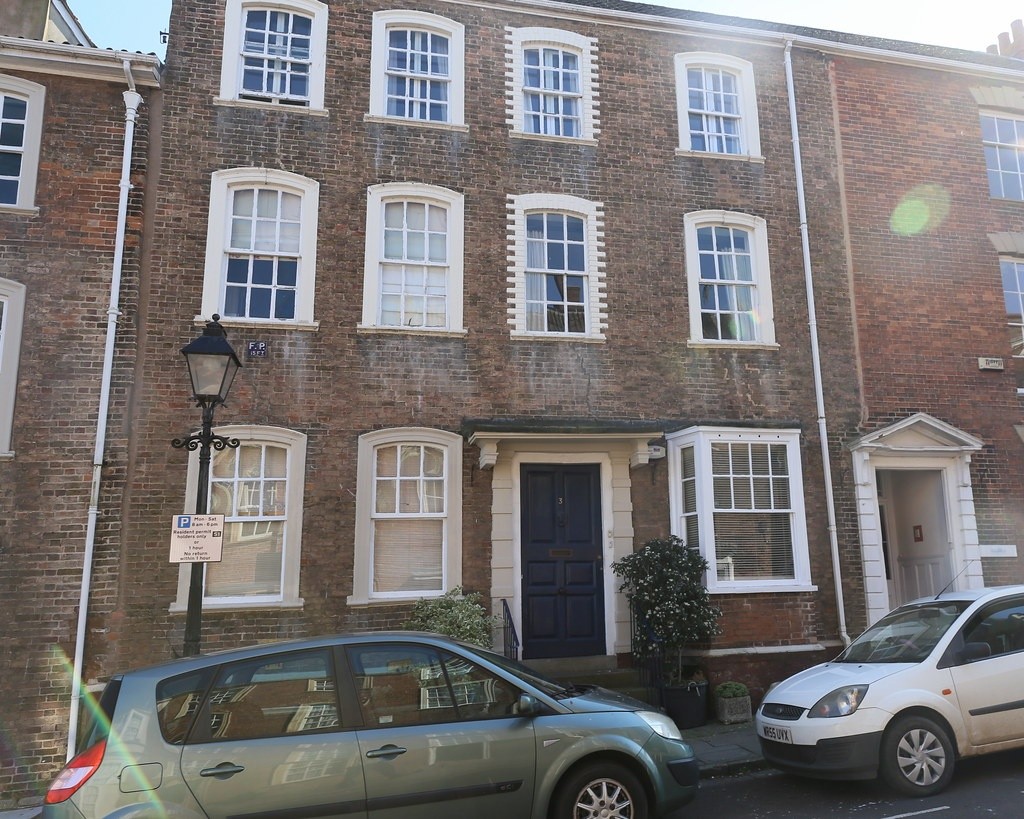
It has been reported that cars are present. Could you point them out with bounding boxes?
[44,629,699,818]
[756,585,1024,798]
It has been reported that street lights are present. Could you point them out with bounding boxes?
[171,313,245,660]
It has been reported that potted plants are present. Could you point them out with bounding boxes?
[713,681,752,725]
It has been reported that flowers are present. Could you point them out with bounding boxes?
[610,535,724,685]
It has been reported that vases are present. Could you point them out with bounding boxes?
[661,681,708,729]
